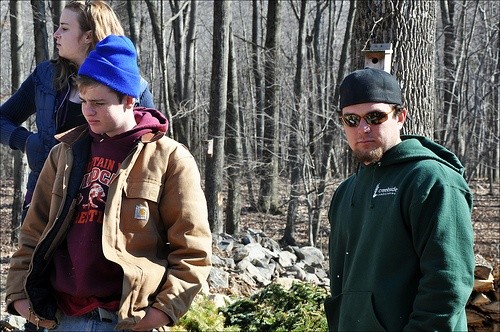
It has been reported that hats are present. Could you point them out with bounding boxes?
[78,34,148,98]
[339,67,402,109]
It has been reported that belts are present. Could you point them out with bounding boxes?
[83,307,115,322]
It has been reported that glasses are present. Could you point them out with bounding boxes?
[342,108,395,127]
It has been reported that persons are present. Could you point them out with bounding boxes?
[5,35,212,332]
[324,66,474,332]
[0,0,156,226]
